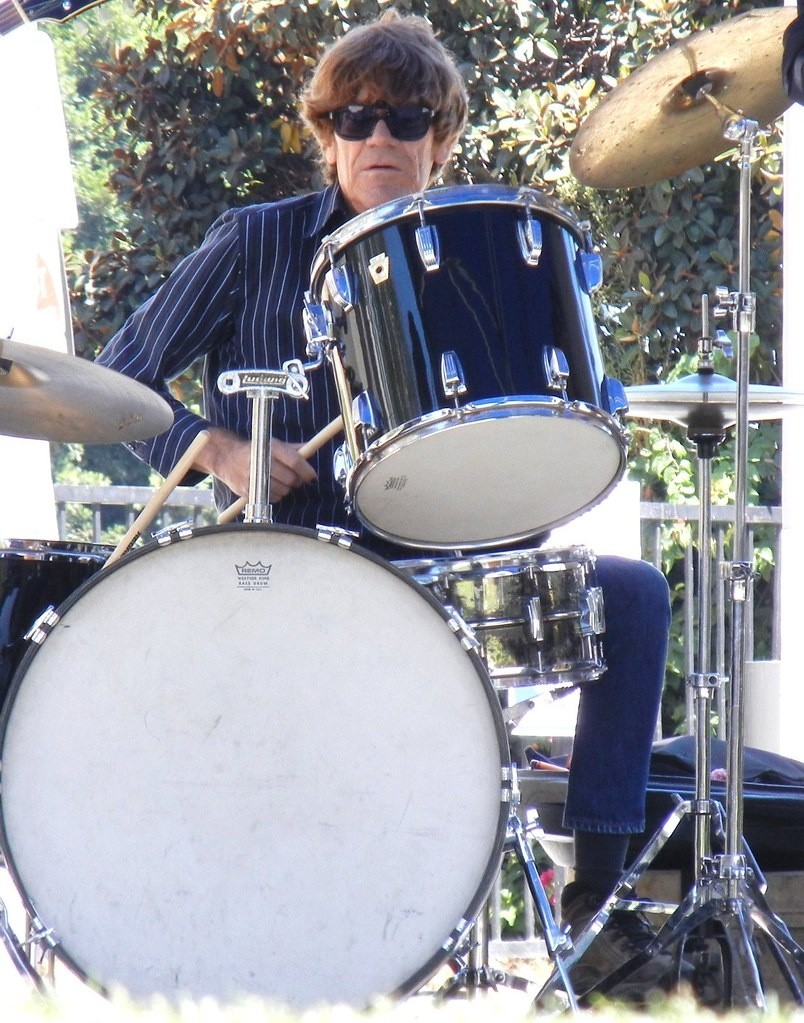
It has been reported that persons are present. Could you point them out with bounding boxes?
[92,12,694,997]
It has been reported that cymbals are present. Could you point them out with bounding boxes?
[569,8,800,191]
[0,337,175,444]
[623,371,783,427]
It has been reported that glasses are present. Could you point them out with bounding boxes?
[324,104,437,142]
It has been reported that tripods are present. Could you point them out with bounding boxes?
[527,91,803,1018]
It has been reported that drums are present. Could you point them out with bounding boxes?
[304,178,630,553]
[387,543,608,688]
[0,524,520,1003]
[0,530,139,714]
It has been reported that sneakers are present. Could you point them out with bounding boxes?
[553,879,694,1000]
